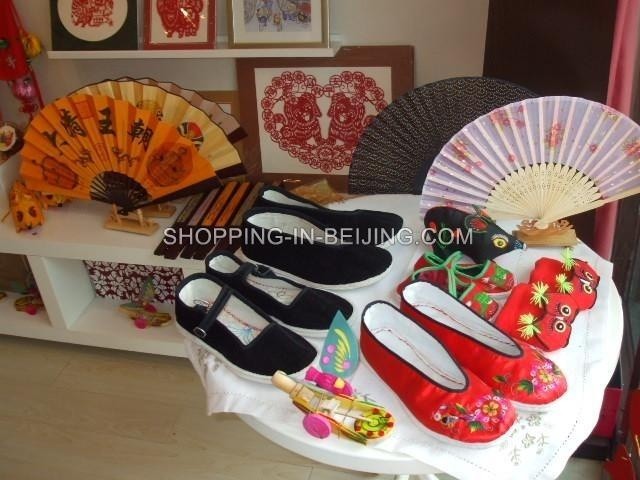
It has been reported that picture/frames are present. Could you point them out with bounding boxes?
[234,44,416,184]
[50,0,137,50]
[144,1,214,50]
[225,0,329,48]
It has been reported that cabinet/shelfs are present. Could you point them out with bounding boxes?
[1,152,206,358]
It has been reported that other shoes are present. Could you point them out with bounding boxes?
[175,189,600,444]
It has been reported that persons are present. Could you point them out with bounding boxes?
[270,366,395,445]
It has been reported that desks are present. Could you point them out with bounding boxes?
[185,193,626,480]
